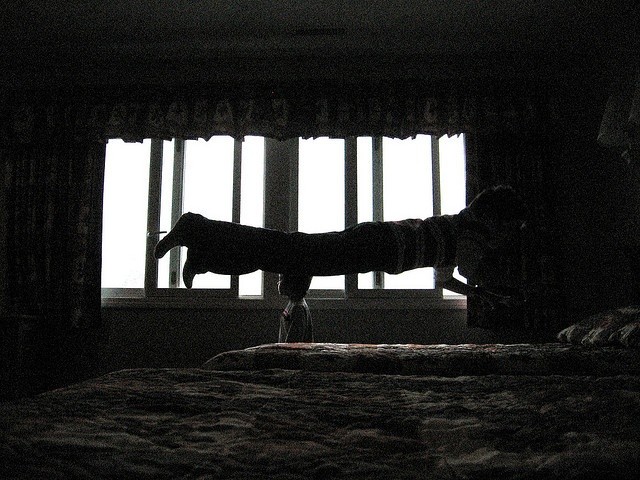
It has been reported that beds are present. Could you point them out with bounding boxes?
[0,367,639,479]
[201,302,639,376]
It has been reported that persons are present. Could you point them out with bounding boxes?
[277,272,314,343]
[155,183,537,309]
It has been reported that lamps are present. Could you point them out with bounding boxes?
[598,86,632,171]
[626,69,640,149]
